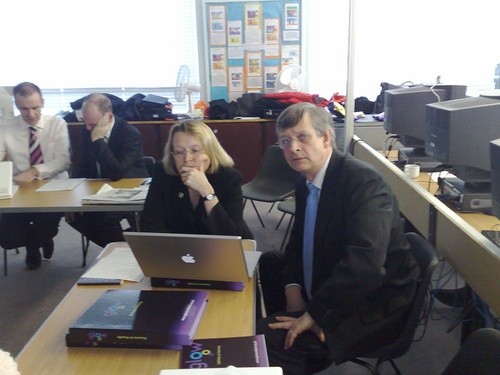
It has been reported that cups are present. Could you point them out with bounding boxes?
[404,164,420,179]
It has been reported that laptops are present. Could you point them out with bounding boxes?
[122,230,264,283]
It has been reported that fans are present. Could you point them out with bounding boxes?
[273,65,307,95]
[174,64,201,118]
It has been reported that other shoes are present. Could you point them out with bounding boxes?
[41,238,54,259]
[26,243,42,270]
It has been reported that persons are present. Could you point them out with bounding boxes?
[0,82,71,270]
[256,102,420,375]
[67,94,147,249]
[143,118,263,333]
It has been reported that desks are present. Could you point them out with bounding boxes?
[14,239,256,375]
[65,119,280,185]
[0,178,152,232]
[351,136,500,320]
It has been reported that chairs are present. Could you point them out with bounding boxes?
[243,145,500,375]
[80,156,156,270]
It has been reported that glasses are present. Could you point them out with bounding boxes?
[170,147,203,158]
[278,131,316,149]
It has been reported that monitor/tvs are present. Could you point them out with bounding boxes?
[489,137,500,220]
[424,94,500,182]
[382,82,467,148]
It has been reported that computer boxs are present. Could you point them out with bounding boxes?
[438,176,492,212]
[395,145,447,172]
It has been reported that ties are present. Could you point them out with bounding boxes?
[28,128,43,165]
[303,183,321,299]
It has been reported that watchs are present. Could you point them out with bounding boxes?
[203,194,216,201]
